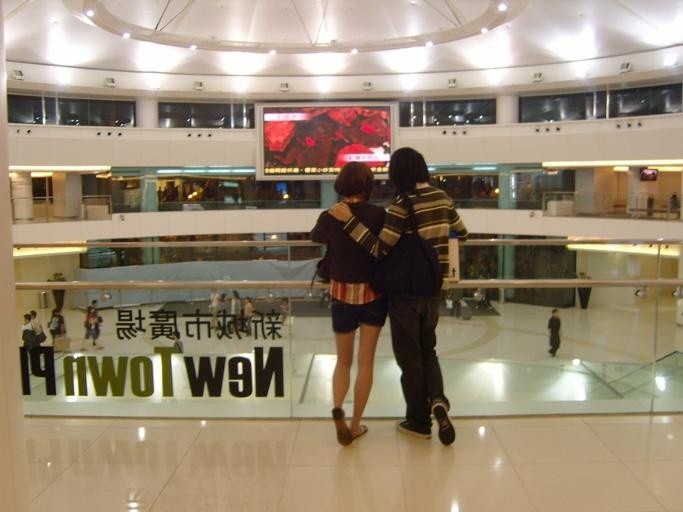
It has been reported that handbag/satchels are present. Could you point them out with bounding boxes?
[368,231,444,300]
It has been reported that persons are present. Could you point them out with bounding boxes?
[326,145,469,446]
[47,309,65,352]
[281,297,290,331]
[30,310,48,369]
[447,286,494,320]
[173,331,184,353]
[21,312,39,376]
[244,296,255,336]
[231,289,242,330]
[79,299,105,352]
[208,286,221,328]
[671,191,680,209]
[311,159,386,446]
[218,291,233,334]
[548,308,561,357]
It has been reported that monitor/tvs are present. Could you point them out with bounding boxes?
[640,169,658,182]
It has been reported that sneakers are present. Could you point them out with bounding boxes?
[352,421,369,440]
[330,406,352,448]
[396,419,432,439]
[430,398,457,446]
